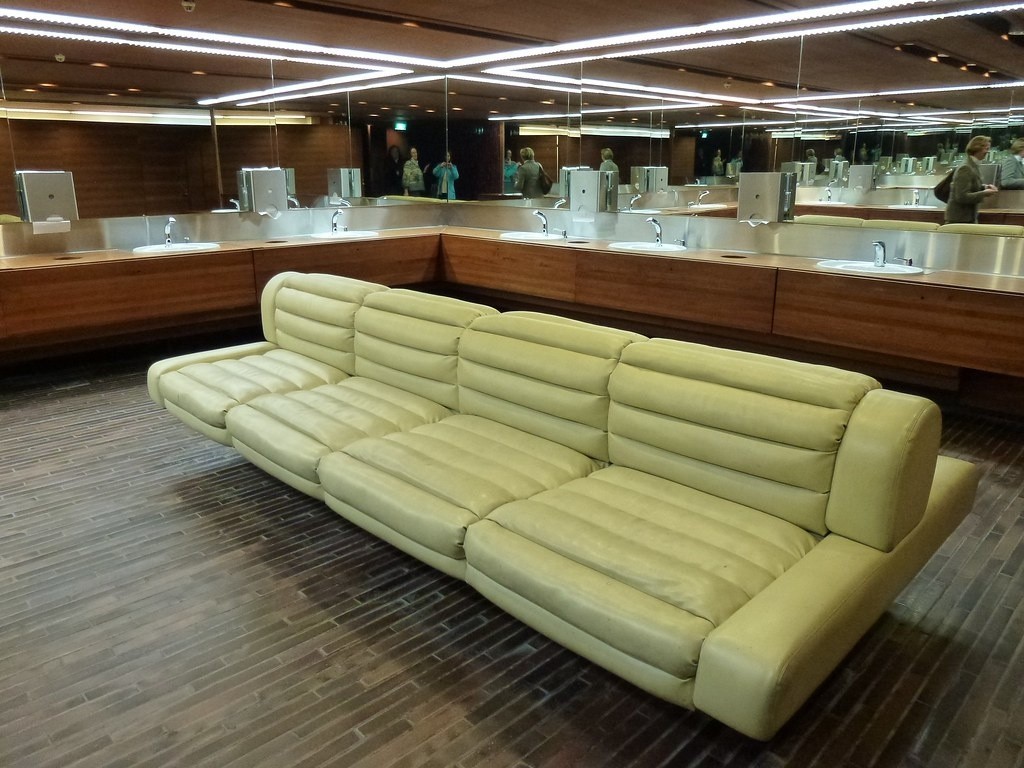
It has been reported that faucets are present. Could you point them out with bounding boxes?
[629,194,642,209]
[909,188,919,205]
[553,199,567,208]
[646,216,662,247]
[826,179,836,201]
[287,195,302,208]
[339,198,352,207]
[332,209,348,232]
[165,216,176,244]
[872,241,887,268]
[229,198,240,211]
[533,210,548,234]
[697,190,709,204]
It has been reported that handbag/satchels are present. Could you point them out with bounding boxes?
[934,169,955,203]
[816,160,825,175]
[537,164,553,196]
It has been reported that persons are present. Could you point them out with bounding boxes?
[860,143,869,160]
[432,151,459,200]
[600,148,618,171]
[834,148,846,161]
[806,148,817,163]
[871,143,880,162]
[995,132,1024,190]
[731,150,743,176]
[504,148,521,194]
[402,146,425,197]
[944,136,998,225]
[384,145,404,196]
[712,148,724,176]
[514,147,544,200]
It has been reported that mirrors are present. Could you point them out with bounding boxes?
[0,0,1024,226]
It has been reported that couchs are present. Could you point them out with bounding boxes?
[147,271,978,740]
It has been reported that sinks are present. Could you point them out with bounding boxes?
[798,200,847,206]
[886,204,938,209]
[816,260,924,275]
[133,243,219,253]
[608,242,687,252]
[620,209,661,214]
[310,230,379,239]
[501,231,564,240]
[688,203,727,209]
